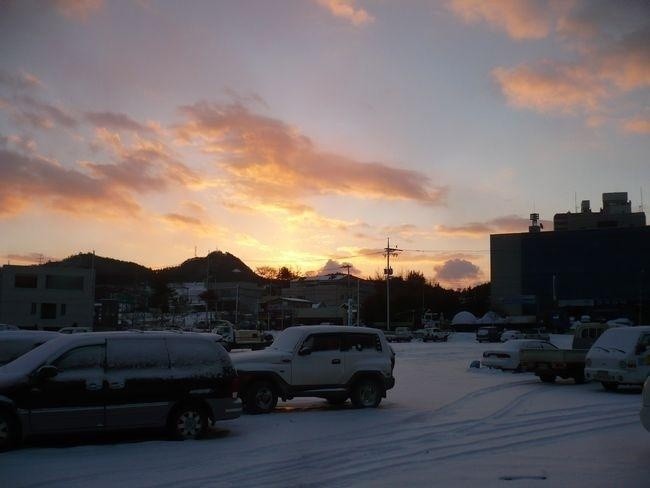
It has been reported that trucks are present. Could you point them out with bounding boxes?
[519,322,630,385]
[422,327,450,342]
[211,323,275,352]
[384,326,414,343]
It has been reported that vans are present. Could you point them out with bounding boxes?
[0,330,244,450]
[476,326,501,343]
[584,325,650,392]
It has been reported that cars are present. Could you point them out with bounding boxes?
[516,327,551,341]
[500,329,526,343]
[482,339,560,373]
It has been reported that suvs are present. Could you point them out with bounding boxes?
[228,325,397,414]
